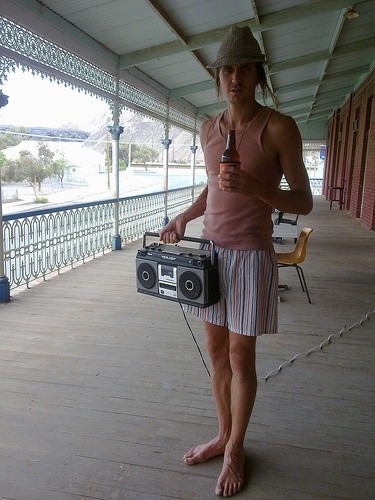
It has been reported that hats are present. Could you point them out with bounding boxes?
[206,25,271,68]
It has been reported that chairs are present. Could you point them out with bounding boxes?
[272,228,313,304]
[329,187,343,210]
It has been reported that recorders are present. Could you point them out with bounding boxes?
[135,232,220,308]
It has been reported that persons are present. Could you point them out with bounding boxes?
[159,25,313,497]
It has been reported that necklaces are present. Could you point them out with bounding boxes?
[224,108,256,152]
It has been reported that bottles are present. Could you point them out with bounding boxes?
[220,129,241,191]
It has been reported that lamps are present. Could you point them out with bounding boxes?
[343,9,359,19]
